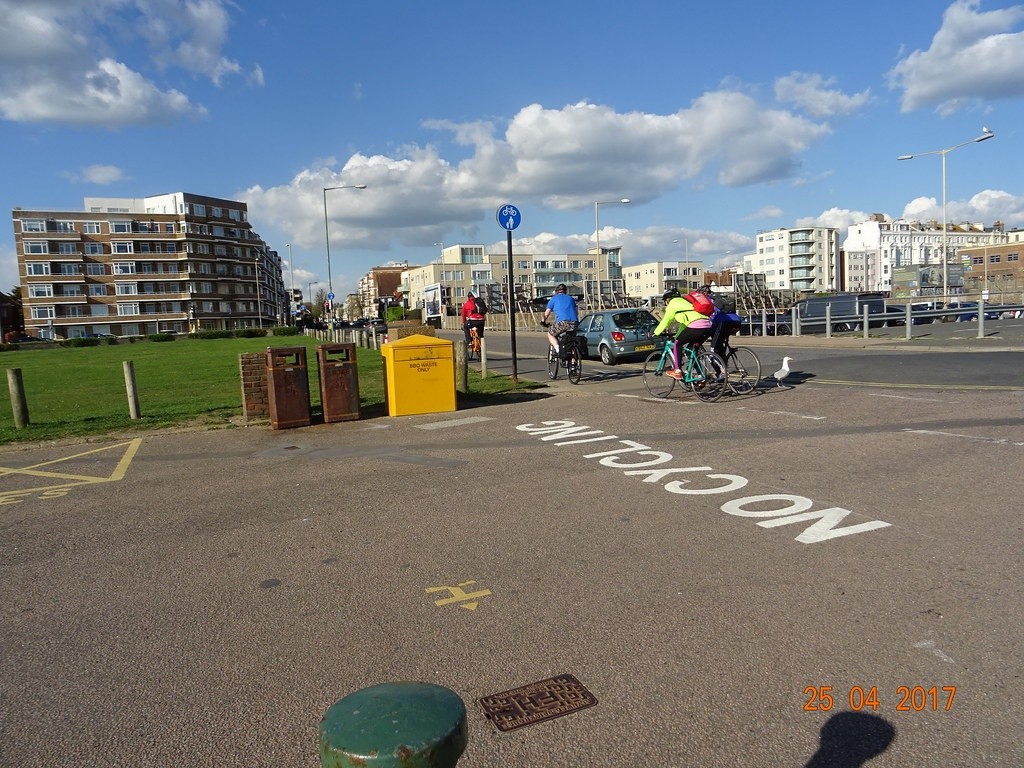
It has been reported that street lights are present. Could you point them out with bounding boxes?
[322,183,367,332]
[255,257,263,330]
[673,238,690,295]
[285,243,295,328]
[718,251,730,296]
[896,132,995,321]
[433,242,447,303]
[594,199,631,310]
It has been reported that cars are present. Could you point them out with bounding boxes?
[366,319,387,336]
[576,306,669,366]
[884,300,1024,326]
[739,313,782,336]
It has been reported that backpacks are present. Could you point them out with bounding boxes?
[707,292,736,314]
[675,291,714,316]
[470,297,487,315]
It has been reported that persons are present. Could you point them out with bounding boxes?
[461,291,486,352]
[695,285,741,383]
[541,284,579,375]
[652,288,713,389]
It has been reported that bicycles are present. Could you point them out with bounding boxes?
[641,330,762,402]
[539,321,582,385]
[461,320,481,360]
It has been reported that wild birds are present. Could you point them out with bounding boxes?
[774,356,794,389]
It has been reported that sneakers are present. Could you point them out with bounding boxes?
[666,371,682,380]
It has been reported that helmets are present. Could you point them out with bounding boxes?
[555,284,567,291]
[468,291,474,297]
[662,289,680,299]
[695,285,711,295]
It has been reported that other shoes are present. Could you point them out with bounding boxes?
[465,341,473,345]
[553,353,560,359]
[570,370,577,375]
[716,372,729,381]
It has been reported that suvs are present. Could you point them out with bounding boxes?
[773,291,887,336]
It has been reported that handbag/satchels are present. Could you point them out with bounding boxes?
[559,336,575,360]
[572,334,589,359]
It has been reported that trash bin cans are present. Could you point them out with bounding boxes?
[380,333,458,417]
[315,342,362,423]
[265,346,312,430]
